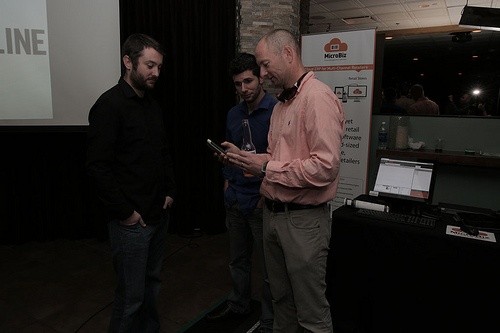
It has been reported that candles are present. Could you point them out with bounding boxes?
[395,125,409,150]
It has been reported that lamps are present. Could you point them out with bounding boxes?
[458,4,500,28]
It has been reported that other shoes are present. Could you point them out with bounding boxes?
[255,318,274,333]
[219,301,259,329]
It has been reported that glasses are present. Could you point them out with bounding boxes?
[276,72,307,105]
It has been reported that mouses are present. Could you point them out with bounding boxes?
[460,224,478,235]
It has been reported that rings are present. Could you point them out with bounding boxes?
[242,163,243,165]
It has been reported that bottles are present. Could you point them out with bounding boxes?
[241,119,257,177]
[378,122,387,150]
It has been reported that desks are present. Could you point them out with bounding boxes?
[323,196,500,333]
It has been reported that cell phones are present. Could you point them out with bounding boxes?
[206,139,227,156]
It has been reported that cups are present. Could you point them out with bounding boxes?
[389,116,409,149]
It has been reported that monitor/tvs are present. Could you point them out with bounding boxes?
[372,157,436,205]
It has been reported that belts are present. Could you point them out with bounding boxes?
[264,197,326,213]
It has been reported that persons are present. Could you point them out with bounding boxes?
[380,82,439,115]
[447,94,497,115]
[213,29,345,333]
[207,52,279,333]
[89,34,177,333]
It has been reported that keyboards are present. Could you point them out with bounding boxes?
[353,207,439,228]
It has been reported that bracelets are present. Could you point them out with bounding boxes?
[261,161,269,175]
